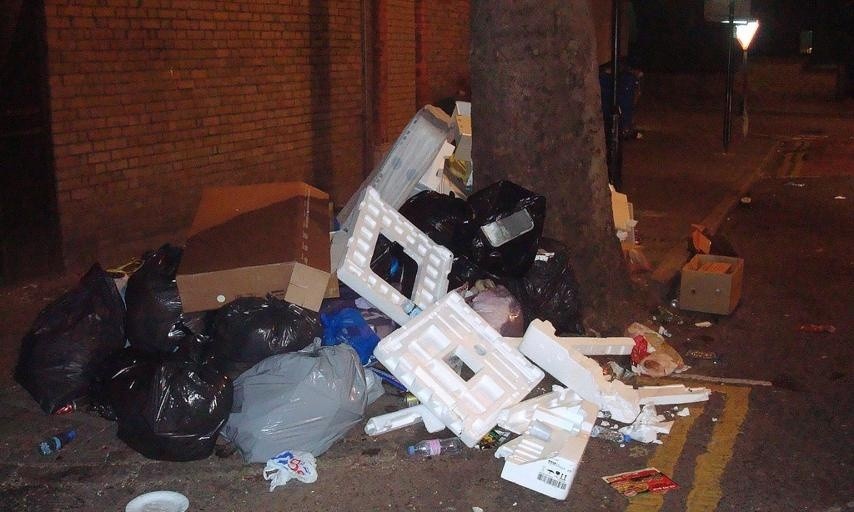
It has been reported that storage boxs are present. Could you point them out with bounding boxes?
[175,180,340,316]
[680,253,745,315]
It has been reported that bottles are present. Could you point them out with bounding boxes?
[32,425,77,457]
[407,436,465,457]
[590,426,631,443]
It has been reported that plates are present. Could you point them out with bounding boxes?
[125,491,189,512]
